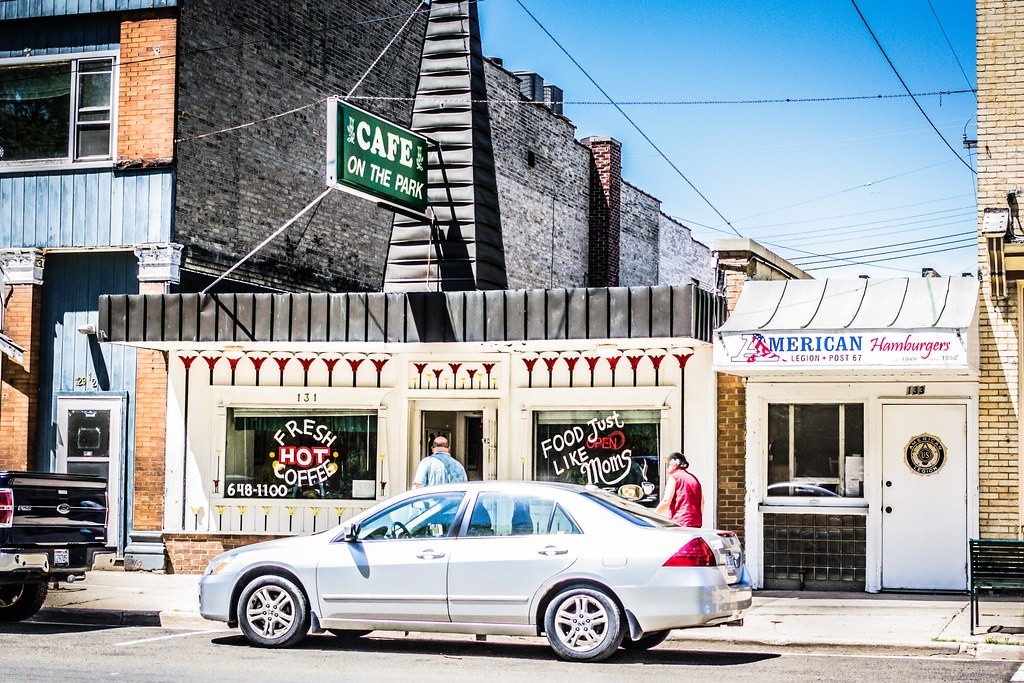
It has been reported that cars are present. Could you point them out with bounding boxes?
[768,482,842,498]
[196,478,754,663]
[625,455,660,483]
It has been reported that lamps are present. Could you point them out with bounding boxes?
[77,324,96,335]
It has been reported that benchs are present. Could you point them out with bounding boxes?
[968,538,1024,635]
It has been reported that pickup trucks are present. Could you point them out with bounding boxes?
[0,469,119,626]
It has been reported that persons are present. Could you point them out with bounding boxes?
[412,436,468,490]
[653,452,705,529]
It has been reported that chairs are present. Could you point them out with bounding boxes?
[511,510,533,535]
[467,504,494,536]
